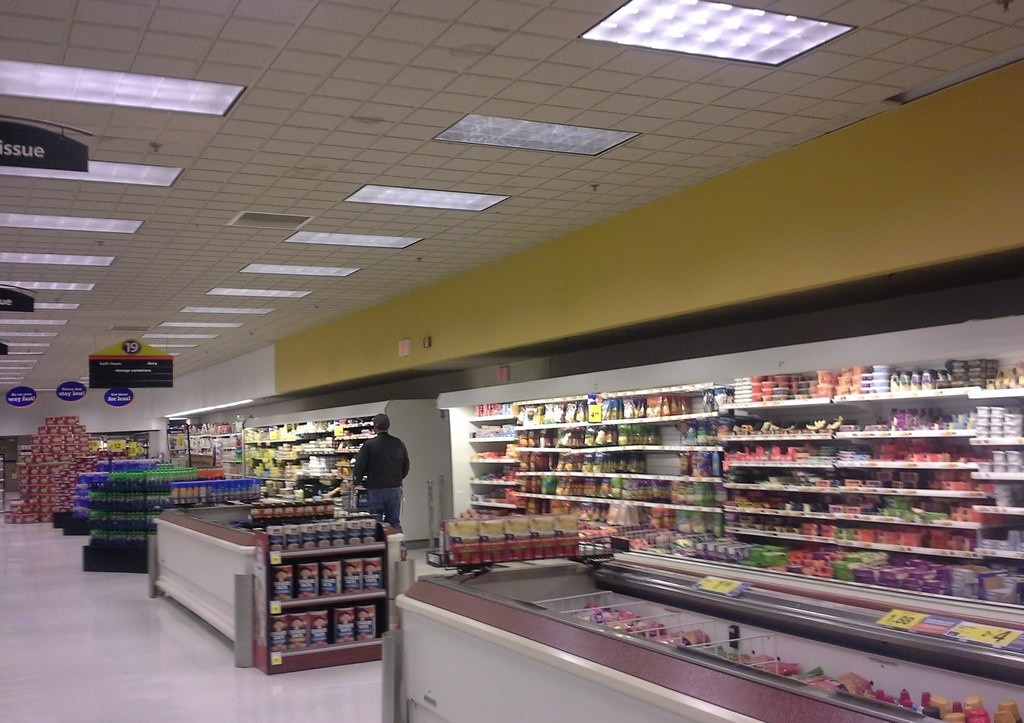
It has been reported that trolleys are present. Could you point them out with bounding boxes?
[333,482,370,519]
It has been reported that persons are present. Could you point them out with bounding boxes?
[352,413,410,561]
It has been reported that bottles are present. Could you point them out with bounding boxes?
[72,461,225,544]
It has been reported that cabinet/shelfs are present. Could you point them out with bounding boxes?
[395,383,1023,723]
[145,400,450,676]
